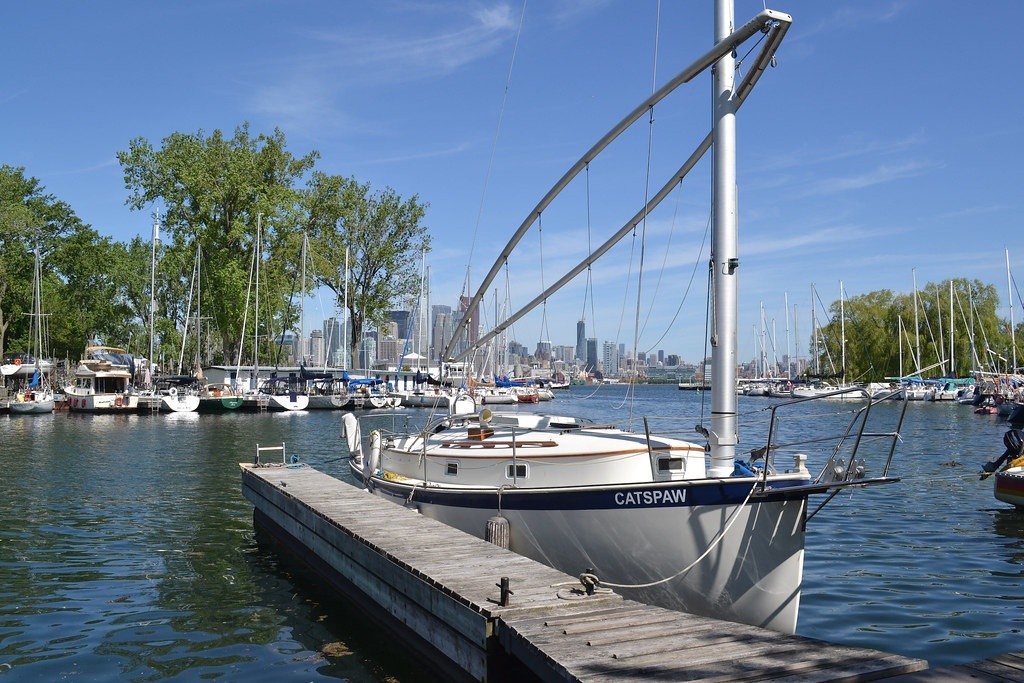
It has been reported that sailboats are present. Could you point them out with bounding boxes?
[339,0,908,637]
[0,206,573,412]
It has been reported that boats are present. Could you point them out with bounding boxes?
[992,424,1024,513]
[678,245,1024,413]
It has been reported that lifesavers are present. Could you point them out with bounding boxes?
[115,398,122,406]
[368,429,380,473]
[169,387,177,397]
